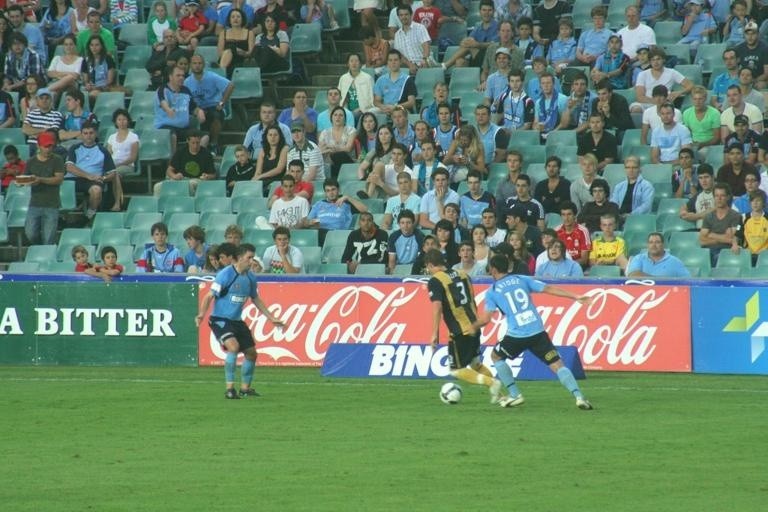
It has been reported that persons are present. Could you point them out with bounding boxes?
[1,0,768,283]
[424,248,505,406]
[194,242,285,399]
[469,256,596,410]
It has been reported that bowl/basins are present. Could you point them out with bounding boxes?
[16,176,33,183]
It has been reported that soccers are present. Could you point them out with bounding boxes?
[439,383,463,405]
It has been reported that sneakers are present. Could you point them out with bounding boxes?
[497,392,526,408]
[575,395,594,410]
[222,387,245,400]
[488,378,503,405]
[239,388,262,398]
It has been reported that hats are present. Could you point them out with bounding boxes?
[37,132,56,148]
[34,87,54,98]
[501,200,530,219]
[494,45,512,58]
[606,33,624,41]
[743,20,759,32]
[184,0,201,8]
[688,0,706,6]
[734,114,750,124]
[290,120,305,132]
